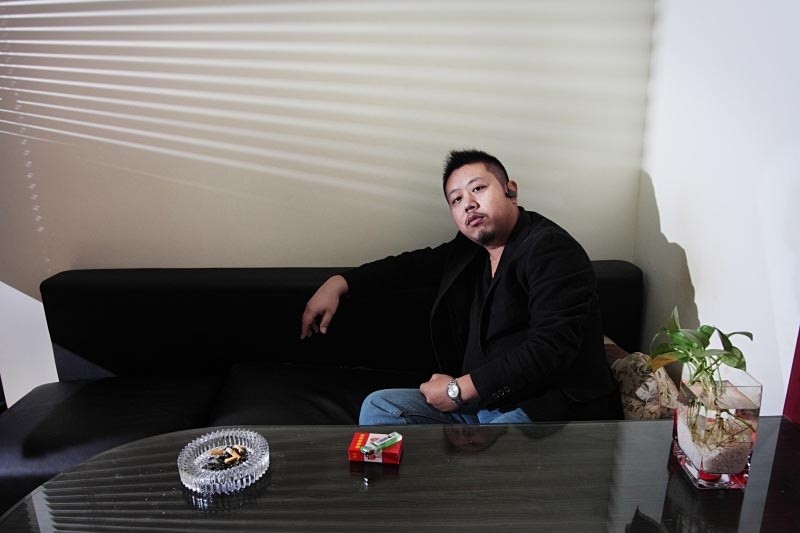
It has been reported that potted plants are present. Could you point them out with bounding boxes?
[646,304,763,490]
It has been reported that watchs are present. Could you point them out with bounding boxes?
[447,377,463,409]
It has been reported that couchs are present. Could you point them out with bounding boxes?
[1,257,644,514]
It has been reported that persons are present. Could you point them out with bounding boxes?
[301,150,625,426]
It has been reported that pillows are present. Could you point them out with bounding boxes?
[610,350,679,420]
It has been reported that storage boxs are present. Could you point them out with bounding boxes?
[348,432,404,465]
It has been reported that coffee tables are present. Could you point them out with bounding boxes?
[1,413,800,533]
